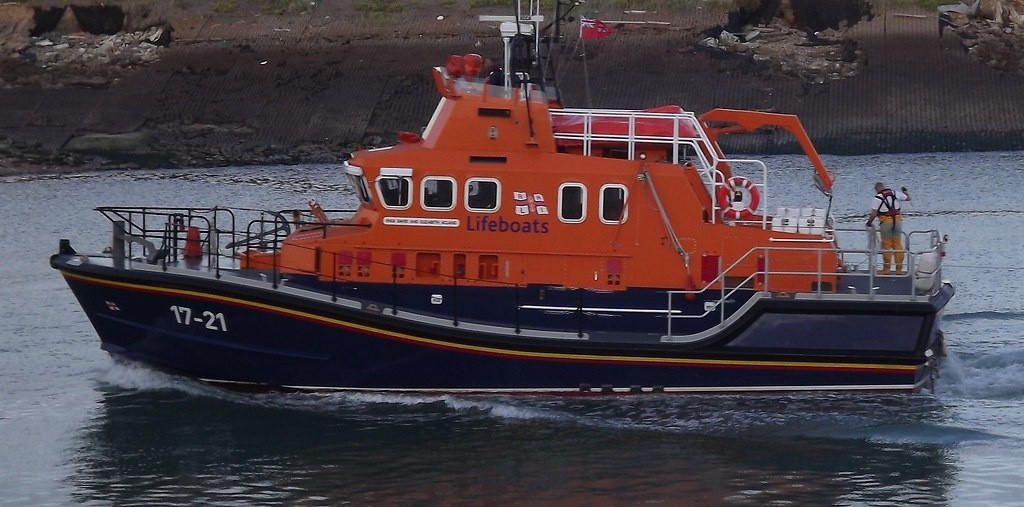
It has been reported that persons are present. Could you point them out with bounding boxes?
[865,181,911,275]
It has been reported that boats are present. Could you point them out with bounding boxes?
[51,2,961,391]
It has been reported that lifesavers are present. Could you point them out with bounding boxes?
[718,177,761,218]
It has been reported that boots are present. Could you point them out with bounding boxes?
[896,260,903,275]
[880,260,891,275]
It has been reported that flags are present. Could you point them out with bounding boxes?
[582,18,616,40]
